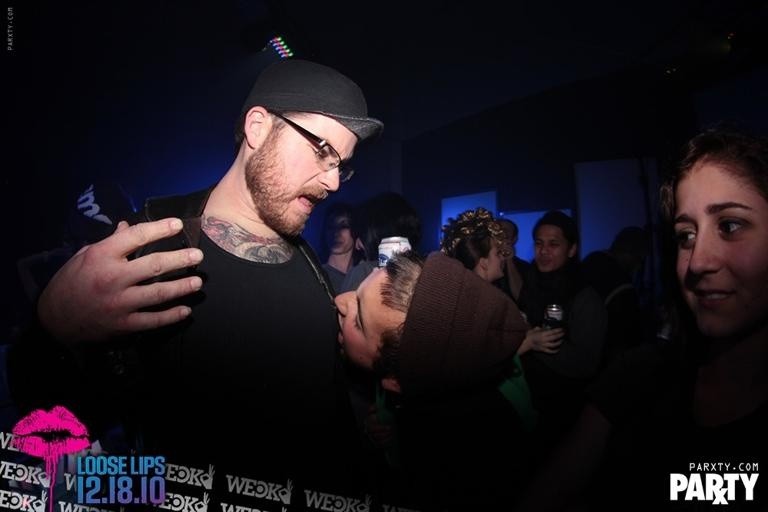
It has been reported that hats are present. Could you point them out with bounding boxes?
[398,248,531,390]
[242,60,384,144]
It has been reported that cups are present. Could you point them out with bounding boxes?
[543,304,564,350]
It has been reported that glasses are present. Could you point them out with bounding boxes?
[268,108,356,183]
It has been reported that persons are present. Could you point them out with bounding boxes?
[0,59,768,512]
